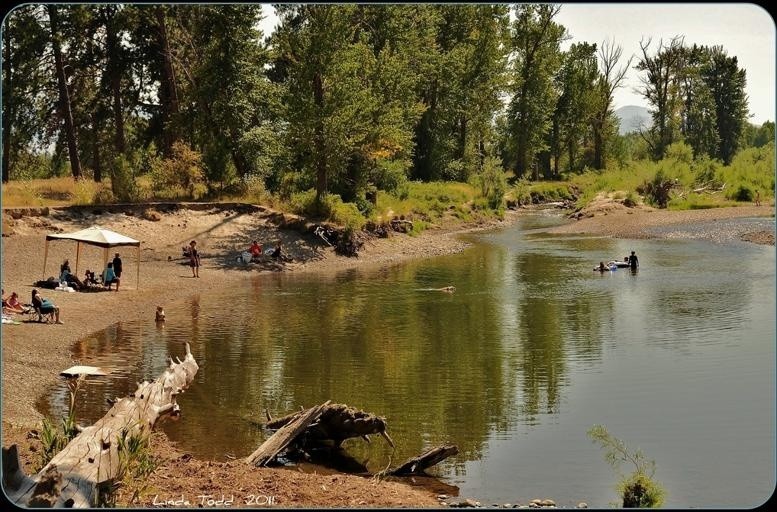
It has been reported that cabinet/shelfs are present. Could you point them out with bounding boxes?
[89,272,101,292]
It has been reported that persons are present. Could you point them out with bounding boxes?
[754,190,760,206]
[155,305,165,321]
[190,294,201,328]
[249,241,261,259]
[61,266,84,288]
[188,241,200,278]
[4,292,27,311]
[622,256,629,262]
[593,261,609,271]
[627,251,637,269]
[105,262,120,291]
[438,285,455,294]
[62,259,71,272]
[252,275,265,306]
[1,288,30,309]
[273,271,287,308]
[112,252,121,279]
[31,289,63,323]
[271,240,292,261]
